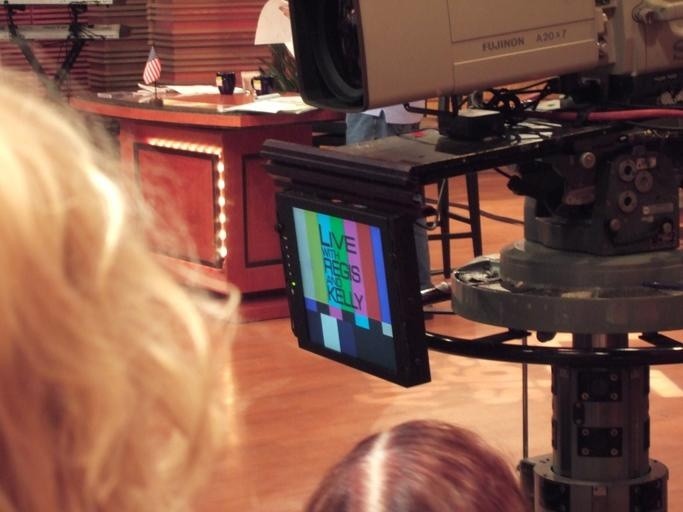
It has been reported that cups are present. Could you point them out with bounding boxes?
[216,71,236,96]
[251,76,274,99]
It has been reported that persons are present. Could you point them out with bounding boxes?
[306,415,533,511]
[0,66,243,512]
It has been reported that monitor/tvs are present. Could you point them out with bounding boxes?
[273,188,432,388]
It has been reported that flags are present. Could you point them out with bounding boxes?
[141,46,162,85]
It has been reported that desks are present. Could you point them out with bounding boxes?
[67,85,346,324]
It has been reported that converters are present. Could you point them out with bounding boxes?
[437,108,503,142]
